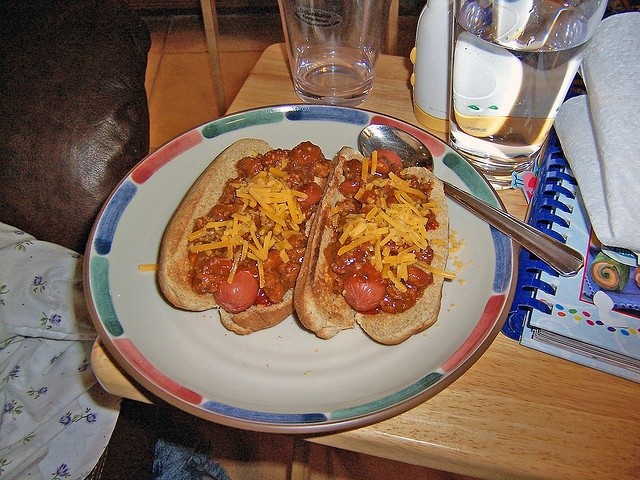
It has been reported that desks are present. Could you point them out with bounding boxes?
[87,42,638,480]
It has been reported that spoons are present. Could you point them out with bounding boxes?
[355,119,588,280]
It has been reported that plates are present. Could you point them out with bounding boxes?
[83,100,521,437]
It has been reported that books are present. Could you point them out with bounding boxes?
[502,124,640,385]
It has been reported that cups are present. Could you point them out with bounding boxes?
[275,0,386,107]
[447,1,609,190]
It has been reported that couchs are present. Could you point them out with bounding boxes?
[1,0,156,479]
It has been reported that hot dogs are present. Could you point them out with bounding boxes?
[293,146,450,346]
[157,139,334,335]
